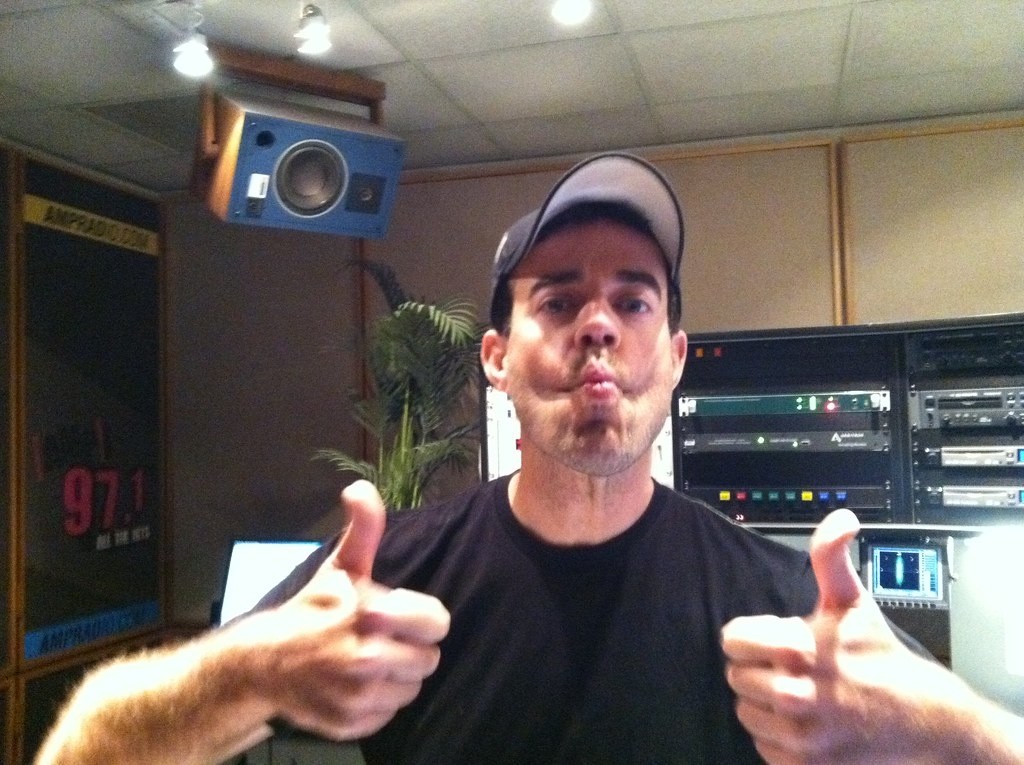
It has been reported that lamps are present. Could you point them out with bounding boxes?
[295,5,332,55]
[172,32,212,79]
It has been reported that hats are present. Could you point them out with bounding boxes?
[486,148,686,334]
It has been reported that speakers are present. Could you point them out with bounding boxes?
[193,89,406,238]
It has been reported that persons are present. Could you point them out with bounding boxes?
[29,150,1023,765]
